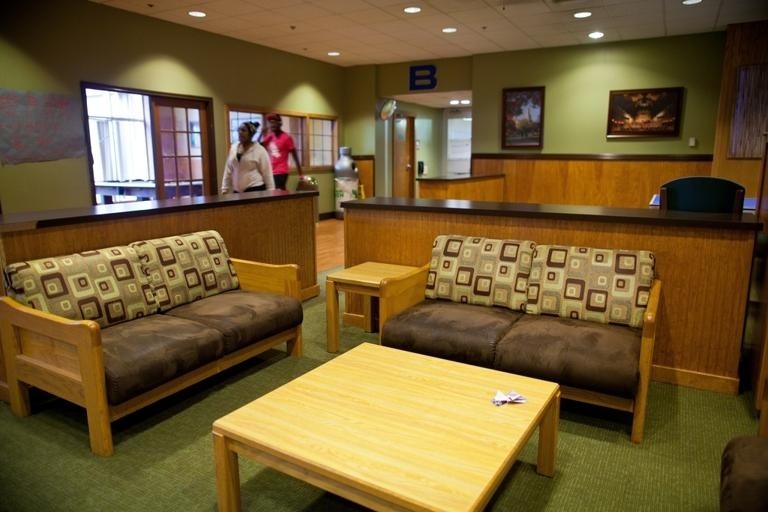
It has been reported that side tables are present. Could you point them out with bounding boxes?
[325,261,421,353]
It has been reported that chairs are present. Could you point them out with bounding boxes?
[658,175,745,214]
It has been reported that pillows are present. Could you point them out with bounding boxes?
[525,244,656,330]
[424,234,537,315]
[1,245,157,329]
[128,229,239,313]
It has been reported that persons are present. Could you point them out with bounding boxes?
[256,113,302,189]
[221,121,276,196]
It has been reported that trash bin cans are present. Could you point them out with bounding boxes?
[334,147,360,220]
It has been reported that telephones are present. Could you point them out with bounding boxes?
[418,161,423,174]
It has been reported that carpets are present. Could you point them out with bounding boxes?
[0,264,760,512]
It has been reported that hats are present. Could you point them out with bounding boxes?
[246,122,259,136]
[267,113,281,121]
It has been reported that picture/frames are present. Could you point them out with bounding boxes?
[606,86,685,139]
[501,85,545,151]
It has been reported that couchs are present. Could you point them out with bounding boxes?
[0,258,303,457]
[378,262,662,444]
[720,399,768,512]
